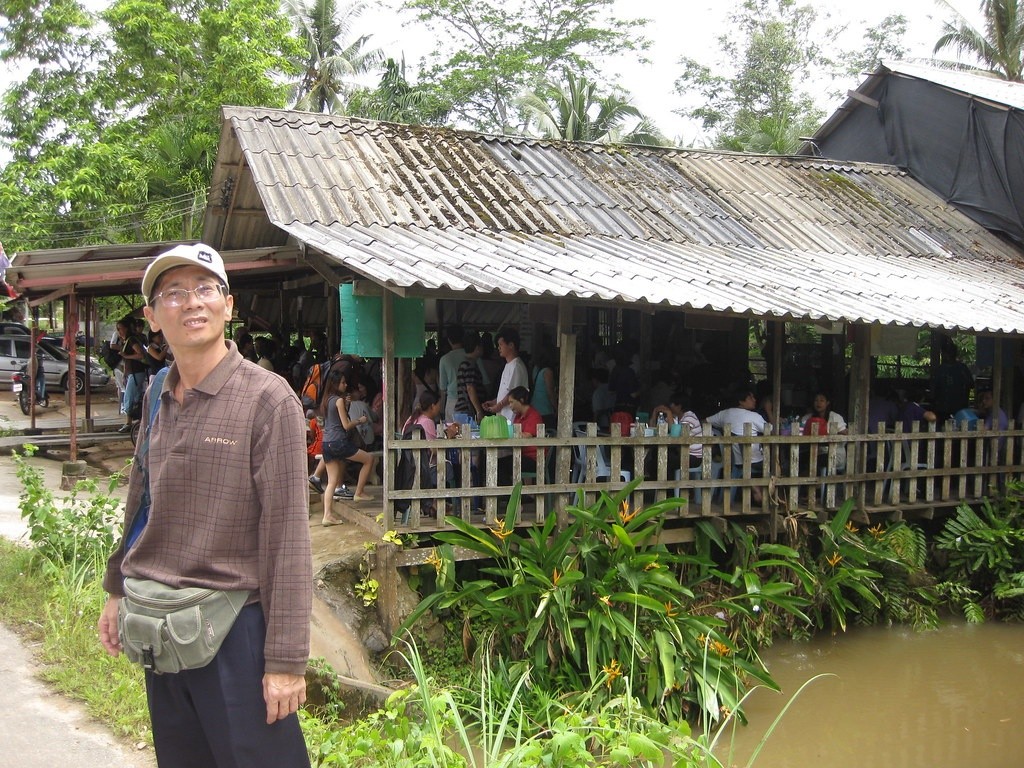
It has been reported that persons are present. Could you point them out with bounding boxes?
[95,244,313,768]
[109,316,174,434]
[232,324,384,527]
[868,341,1009,496]
[571,333,850,506]
[393,326,559,519]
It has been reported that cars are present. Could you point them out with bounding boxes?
[0,321,113,397]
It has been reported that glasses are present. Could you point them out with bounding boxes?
[150,283,227,307]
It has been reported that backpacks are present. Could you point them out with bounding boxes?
[300,354,351,408]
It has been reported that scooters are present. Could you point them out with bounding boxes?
[9,345,49,418]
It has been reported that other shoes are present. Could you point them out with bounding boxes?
[497,505,526,516]
[353,493,377,502]
[474,508,486,515]
[117,424,134,433]
[430,508,454,519]
[322,518,342,527]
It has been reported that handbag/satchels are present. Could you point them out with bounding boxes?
[116,577,254,675]
[100,337,122,370]
[110,366,171,558]
[122,335,153,366]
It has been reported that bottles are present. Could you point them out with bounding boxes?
[635,417,640,436]
[658,412,664,425]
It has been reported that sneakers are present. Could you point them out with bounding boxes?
[308,474,324,494]
[332,484,355,500]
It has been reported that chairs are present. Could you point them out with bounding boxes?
[395,434,460,525]
[515,418,859,511]
[878,426,932,499]
[113,368,127,415]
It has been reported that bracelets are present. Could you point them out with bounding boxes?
[357,419,362,424]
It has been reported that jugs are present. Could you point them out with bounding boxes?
[452,413,472,427]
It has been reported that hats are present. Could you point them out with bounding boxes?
[141,243,229,306]
